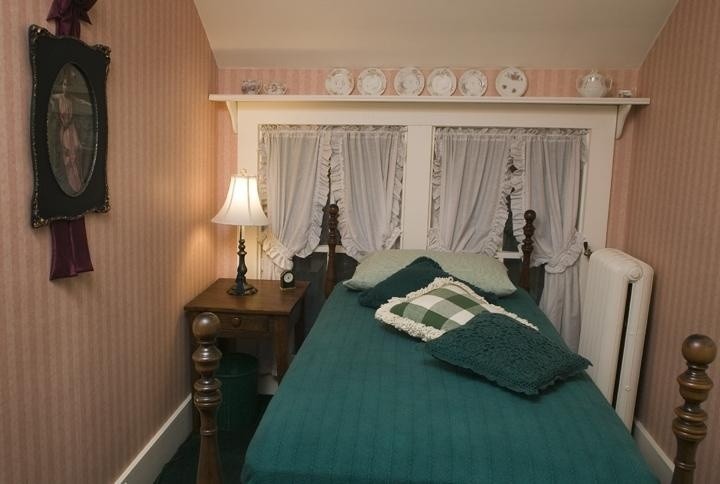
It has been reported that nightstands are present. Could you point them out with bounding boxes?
[180,275,313,435]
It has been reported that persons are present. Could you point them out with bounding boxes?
[48,75,92,193]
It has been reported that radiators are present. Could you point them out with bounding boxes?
[570,244,659,437]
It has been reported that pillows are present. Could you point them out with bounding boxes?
[420,306,594,397]
[373,274,541,344]
[357,256,499,306]
[338,245,519,299]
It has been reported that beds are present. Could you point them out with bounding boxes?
[186,198,719,482]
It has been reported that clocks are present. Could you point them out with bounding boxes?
[279,268,296,290]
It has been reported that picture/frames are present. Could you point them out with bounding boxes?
[22,21,116,231]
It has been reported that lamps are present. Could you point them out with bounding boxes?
[206,169,271,298]
